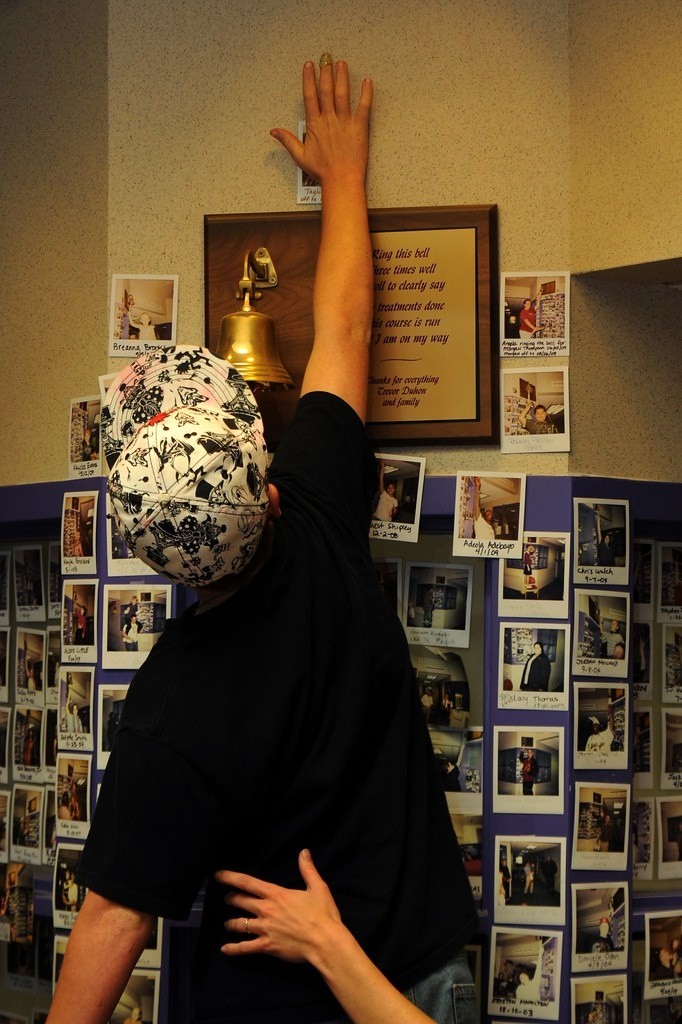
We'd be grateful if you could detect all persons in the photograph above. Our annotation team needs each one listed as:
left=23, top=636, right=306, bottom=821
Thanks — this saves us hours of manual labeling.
left=0, top=293, right=682, bottom=1024
left=41, top=52, right=480, bottom=1024
left=215, top=848, right=439, bottom=1024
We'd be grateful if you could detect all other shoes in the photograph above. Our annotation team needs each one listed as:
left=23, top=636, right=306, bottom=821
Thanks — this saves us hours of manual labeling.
left=424, top=620, right=431, bottom=625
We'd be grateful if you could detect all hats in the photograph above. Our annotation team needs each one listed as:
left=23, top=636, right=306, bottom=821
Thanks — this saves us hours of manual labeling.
left=100, top=345, right=269, bottom=588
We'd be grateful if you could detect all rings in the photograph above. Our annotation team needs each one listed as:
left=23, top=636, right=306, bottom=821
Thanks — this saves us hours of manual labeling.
left=245, top=918, right=251, bottom=933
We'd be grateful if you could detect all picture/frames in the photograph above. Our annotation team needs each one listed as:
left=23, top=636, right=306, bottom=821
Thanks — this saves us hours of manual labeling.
left=204, top=204, right=501, bottom=450
